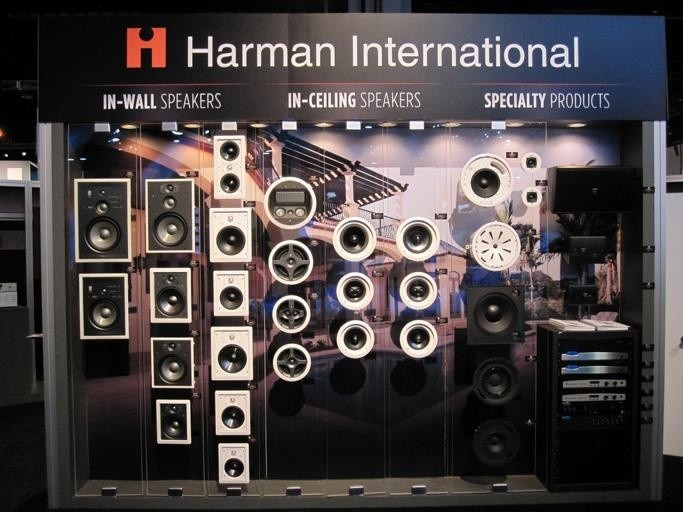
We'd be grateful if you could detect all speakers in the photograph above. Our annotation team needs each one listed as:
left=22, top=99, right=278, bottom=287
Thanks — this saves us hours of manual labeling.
left=453, top=154, right=537, bottom=478
left=74, top=178, right=132, bottom=262
left=78, top=272, right=130, bottom=341
left=265, top=176, right=448, bottom=477
left=144, top=178, right=195, bottom=446
left=535, top=166, right=644, bottom=493
left=208, top=135, right=254, bottom=488
